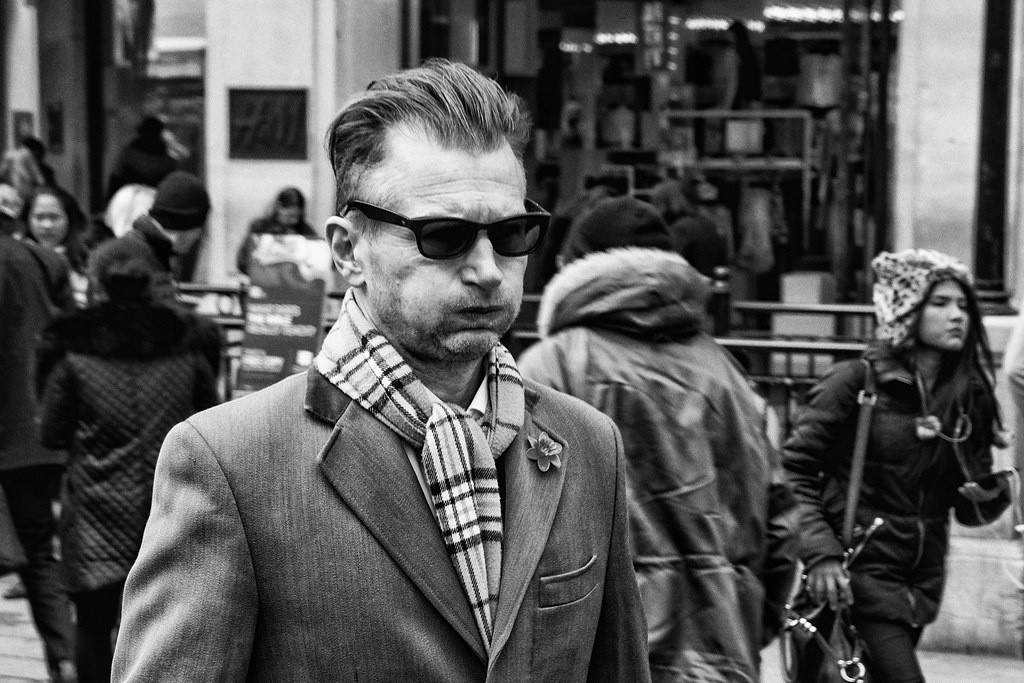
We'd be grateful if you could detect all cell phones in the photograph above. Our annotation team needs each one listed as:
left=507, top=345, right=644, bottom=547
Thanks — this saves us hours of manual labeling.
left=974, top=468, right=1021, bottom=490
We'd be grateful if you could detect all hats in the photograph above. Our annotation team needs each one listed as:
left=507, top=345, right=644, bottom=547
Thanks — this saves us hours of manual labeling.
left=563, top=196, right=672, bottom=263
left=136, top=115, right=163, bottom=139
left=872, top=249, right=1015, bottom=448
left=149, top=171, right=208, bottom=228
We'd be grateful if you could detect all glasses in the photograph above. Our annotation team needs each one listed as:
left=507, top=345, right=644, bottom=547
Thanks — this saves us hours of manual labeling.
left=336, top=198, right=552, bottom=260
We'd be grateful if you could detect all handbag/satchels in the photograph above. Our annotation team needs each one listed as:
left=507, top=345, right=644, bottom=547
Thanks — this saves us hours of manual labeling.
left=779, top=576, right=876, bottom=683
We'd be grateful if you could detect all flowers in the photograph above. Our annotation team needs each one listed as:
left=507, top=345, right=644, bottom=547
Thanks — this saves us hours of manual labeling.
left=523, top=431, right=563, bottom=472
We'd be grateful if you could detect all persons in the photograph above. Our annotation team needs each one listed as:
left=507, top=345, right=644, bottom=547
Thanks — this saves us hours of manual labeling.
left=236, top=189, right=314, bottom=276
left=652, top=183, right=728, bottom=335
left=109, top=62, right=652, bottom=683
left=513, top=195, right=802, bottom=683
left=0, top=116, right=221, bottom=683
left=782, top=249, right=1015, bottom=682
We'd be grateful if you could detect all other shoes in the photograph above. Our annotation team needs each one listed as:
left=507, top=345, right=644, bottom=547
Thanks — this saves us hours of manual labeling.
left=53, top=658, right=78, bottom=683
